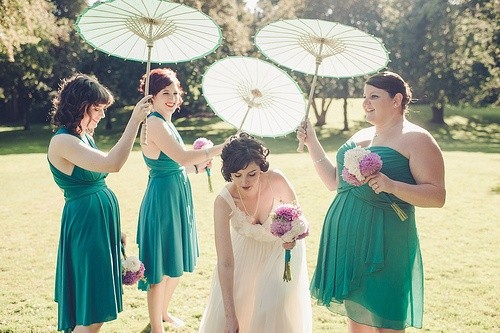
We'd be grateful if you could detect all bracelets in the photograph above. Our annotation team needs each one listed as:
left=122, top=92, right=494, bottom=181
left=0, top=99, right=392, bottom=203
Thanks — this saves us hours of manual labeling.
left=314, top=155, right=326, bottom=164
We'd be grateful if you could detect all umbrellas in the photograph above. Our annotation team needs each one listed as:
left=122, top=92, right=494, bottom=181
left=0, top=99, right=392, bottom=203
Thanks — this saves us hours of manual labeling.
left=200, top=55, right=307, bottom=139
left=73, top=0, right=225, bottom=145
left=251, top=18, right=391, bottom=153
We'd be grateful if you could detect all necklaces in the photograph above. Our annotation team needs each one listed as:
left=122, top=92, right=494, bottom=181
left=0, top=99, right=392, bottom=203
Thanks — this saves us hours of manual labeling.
left=374, top=134, right=379, bottom=137
left=237, top=181, right=261, bottom=217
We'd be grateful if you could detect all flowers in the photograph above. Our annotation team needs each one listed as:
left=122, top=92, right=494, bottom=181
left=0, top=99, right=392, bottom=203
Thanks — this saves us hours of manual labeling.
left=121, top=241, right=146, bottom=286
left=269, top=199, right=310, bottom=283
left=340, top=143, right=409, bottom=220
left=193, top=138, right=216, bottom=195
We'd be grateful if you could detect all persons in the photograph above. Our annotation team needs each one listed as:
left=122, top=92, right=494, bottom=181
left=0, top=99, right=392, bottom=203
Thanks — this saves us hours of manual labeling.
left=198, top=131, right=313, bottom=333
left=297, top=71, right=446, bottom=333
left=47, top=75, right=153, bottom=333
left=136, top=68, right=232, bottom=333
